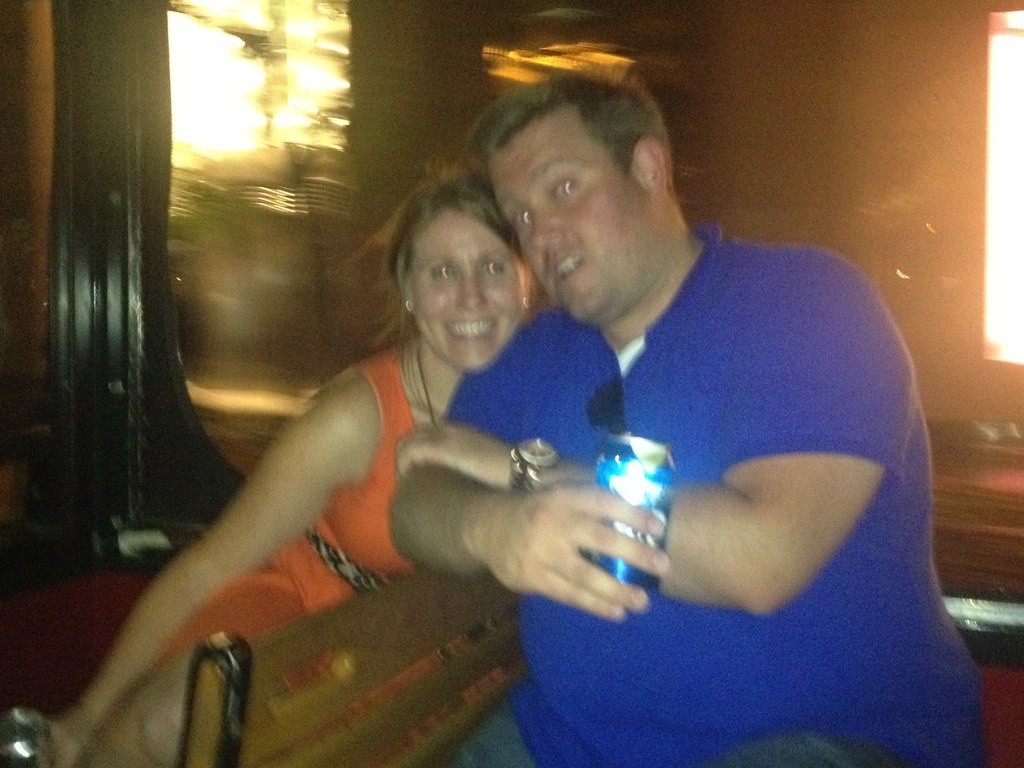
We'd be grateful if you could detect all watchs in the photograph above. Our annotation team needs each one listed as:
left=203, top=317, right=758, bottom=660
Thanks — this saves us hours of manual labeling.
left=509, top=438, right=559, bottom=492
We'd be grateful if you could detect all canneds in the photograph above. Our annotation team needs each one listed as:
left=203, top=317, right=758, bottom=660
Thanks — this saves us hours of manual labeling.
left=0, top=706, right=56, bottom=768
left=586, top=432, right=678, bottom=597
left=508, top=438, right=559, bottom=491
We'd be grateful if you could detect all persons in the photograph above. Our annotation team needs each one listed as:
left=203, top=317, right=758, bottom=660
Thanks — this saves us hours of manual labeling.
left=11, top=160, right=536, bottom=768
left=388, top=69, right=995, bottom=767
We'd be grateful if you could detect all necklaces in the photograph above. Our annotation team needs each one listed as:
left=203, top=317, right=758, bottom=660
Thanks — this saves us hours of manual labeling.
left=417, top=342, right=447, bottom=433
